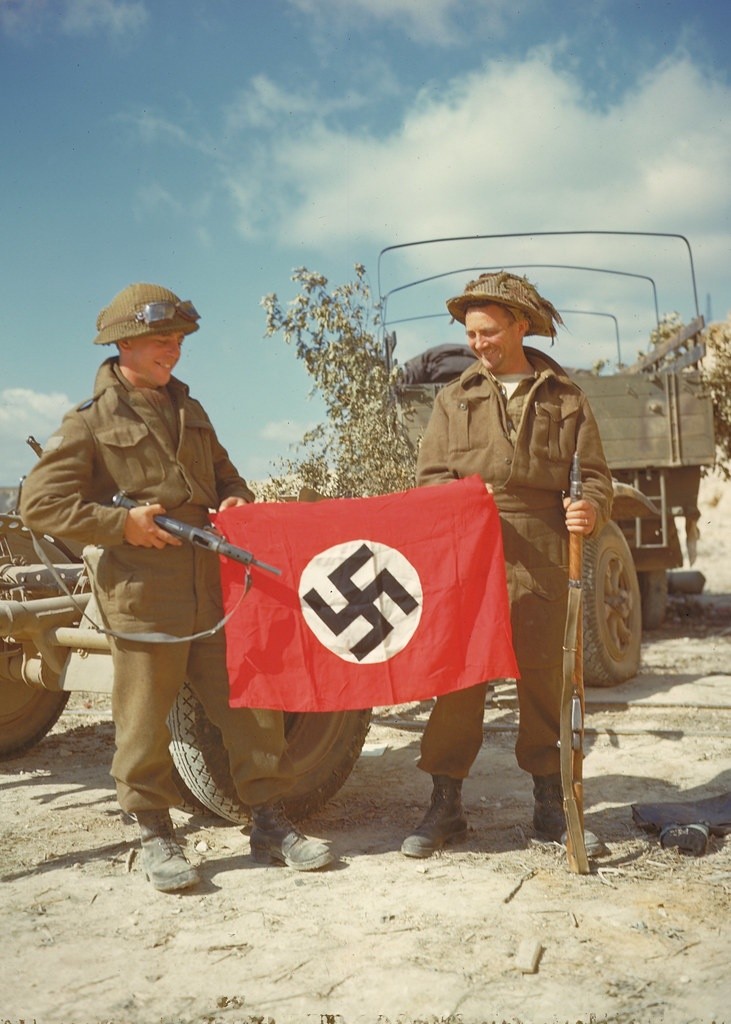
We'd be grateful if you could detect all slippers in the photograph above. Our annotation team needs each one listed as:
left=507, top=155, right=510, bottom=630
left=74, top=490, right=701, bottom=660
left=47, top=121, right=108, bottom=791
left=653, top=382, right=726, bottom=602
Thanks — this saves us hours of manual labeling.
left=532, top=775, right=602, bottom=856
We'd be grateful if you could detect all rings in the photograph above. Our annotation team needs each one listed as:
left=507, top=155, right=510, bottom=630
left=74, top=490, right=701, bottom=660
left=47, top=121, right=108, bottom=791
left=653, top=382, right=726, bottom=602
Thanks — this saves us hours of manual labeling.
left=585, top=518, right=588, bottom=525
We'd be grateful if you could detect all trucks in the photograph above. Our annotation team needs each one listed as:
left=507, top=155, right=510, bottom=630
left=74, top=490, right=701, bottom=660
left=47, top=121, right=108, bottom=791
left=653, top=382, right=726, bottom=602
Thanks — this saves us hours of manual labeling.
left=378, top=230, right=716, bottom=630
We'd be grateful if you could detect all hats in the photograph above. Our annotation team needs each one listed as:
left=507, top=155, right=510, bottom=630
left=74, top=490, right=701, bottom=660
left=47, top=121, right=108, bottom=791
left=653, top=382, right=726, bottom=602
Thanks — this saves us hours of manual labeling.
left=93, top=284, right=200, bottom=344
left=446, top=271, right=556, bottom=337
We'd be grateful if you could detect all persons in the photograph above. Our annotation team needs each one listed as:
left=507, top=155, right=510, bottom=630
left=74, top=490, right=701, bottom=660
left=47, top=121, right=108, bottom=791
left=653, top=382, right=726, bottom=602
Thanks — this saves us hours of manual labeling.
left=401, top=270, right=614, bottom=858
left=21, top=284, right=334, bottom=890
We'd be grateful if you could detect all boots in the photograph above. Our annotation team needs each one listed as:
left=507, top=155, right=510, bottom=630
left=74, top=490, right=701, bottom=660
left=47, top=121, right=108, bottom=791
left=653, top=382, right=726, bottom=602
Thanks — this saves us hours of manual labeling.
left=134, top=809, right=200, bottom=890
left=401, top=774, right=467, bottom=858
left=249, top=797, right=334, bottom=871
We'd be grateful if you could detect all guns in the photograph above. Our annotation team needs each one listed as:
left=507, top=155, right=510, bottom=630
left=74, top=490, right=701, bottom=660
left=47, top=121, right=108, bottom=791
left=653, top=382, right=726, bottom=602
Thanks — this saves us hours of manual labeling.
left=556, top=450, right=591, bottom=876
left=15, top=435, right=284, bottom=578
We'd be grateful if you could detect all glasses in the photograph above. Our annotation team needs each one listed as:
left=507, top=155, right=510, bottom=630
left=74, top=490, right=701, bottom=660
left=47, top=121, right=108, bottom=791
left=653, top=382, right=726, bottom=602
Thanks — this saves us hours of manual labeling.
left=101, top=300, right=201, bottom=331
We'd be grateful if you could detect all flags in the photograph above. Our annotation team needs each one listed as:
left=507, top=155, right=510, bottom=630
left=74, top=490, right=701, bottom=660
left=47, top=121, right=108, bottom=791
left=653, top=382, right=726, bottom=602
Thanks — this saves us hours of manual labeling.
left=207, top=472, right=522, bottom=713
